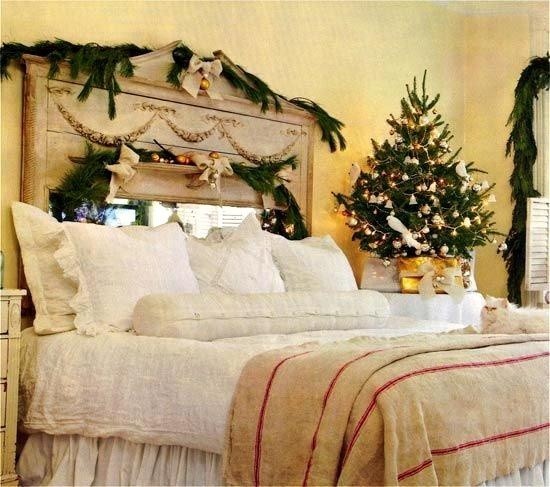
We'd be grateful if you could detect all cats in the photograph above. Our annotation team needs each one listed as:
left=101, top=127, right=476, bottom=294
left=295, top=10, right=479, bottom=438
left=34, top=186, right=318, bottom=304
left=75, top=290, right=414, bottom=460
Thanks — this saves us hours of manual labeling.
left=481, top=294, right=550, bottom=334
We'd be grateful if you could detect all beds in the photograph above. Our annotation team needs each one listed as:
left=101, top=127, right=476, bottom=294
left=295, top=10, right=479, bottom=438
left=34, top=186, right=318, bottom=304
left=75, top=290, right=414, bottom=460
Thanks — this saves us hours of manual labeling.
left=13, top=300, right=550, bottom=485
